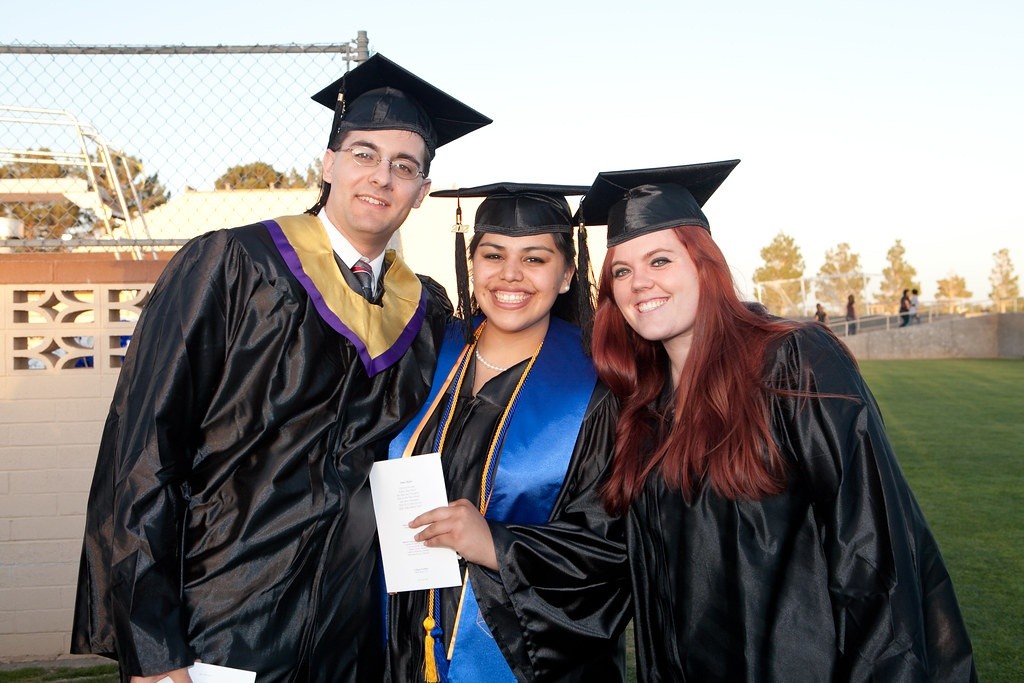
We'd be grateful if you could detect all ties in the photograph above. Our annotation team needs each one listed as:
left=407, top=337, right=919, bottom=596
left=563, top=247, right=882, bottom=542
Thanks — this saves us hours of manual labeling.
left=353, top=260, right=373, bottom=302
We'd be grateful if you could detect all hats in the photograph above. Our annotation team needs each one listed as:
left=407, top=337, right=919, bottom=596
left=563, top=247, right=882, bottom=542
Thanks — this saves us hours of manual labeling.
left=572, top=158, right=741, bottom=358
left=429, top=182, right=591, bottom=346
left=305, top=51, right=493, bottom=217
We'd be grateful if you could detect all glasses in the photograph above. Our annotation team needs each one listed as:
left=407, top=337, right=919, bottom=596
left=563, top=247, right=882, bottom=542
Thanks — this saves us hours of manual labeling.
left=330, top=146, right=426, bottom=179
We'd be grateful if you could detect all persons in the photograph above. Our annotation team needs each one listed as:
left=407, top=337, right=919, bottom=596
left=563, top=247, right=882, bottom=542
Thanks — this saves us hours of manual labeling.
left=71, top=52, right=454, bottom=683
left=815, top=288, right=921, bottom=336
left=570, top=159, right=979, bottom=683
left=388, top=182, right=637, bottom=683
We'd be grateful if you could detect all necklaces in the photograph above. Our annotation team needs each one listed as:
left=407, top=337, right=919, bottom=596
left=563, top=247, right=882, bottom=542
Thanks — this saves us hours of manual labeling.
left=473, top=348, right=509, bottom=375
